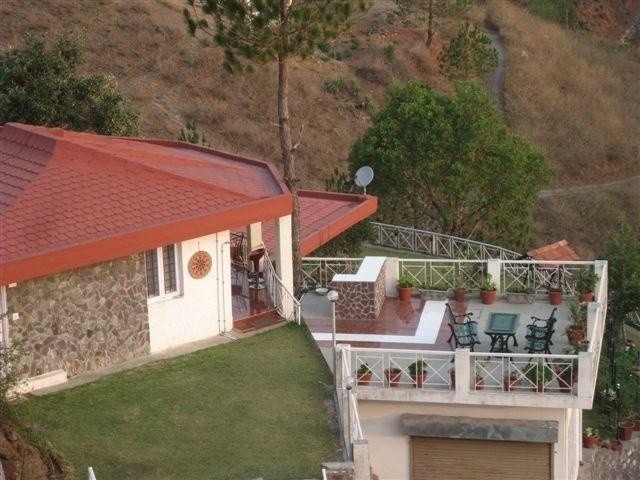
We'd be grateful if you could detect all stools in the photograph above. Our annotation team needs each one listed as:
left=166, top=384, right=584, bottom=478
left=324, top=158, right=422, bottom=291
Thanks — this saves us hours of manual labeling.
left=247, top=248, right=265, bottom=290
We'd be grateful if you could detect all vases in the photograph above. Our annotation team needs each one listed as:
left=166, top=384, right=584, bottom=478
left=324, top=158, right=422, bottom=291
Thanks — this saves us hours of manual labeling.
left=354, top=359, right=430, bottom=389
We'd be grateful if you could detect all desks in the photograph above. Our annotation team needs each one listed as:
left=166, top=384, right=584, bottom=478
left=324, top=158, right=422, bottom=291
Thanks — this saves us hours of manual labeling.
left=483, top=309, right=521, bottom=360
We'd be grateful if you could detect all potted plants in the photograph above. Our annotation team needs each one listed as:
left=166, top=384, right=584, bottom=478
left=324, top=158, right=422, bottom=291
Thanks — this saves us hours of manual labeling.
left=548, top=268, right=600, bottom=394
left=448, top=365, right=484, bottom=391
left=452, top=272, right=498, bottom=305
left=395, top=269, right=416, bottom=302
left=506, top=285, right=537, bottom=305
left=583, top=411, right=640, bottom=456
left=501, top=359, right=552, bottom=393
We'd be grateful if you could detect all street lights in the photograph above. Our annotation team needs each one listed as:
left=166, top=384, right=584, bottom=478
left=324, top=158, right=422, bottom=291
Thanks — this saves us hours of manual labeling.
left=341, top=373, right=356, bottom=462
left=325, top=288, right=339, bottom=397
left=607, top=315, right=622, bottom=440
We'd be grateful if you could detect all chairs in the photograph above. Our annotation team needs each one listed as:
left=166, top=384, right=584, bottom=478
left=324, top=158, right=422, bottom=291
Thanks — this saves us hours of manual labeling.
left=441, top=301, right=482, bottom=364
left=523, top=308, right=559, bottom=368
